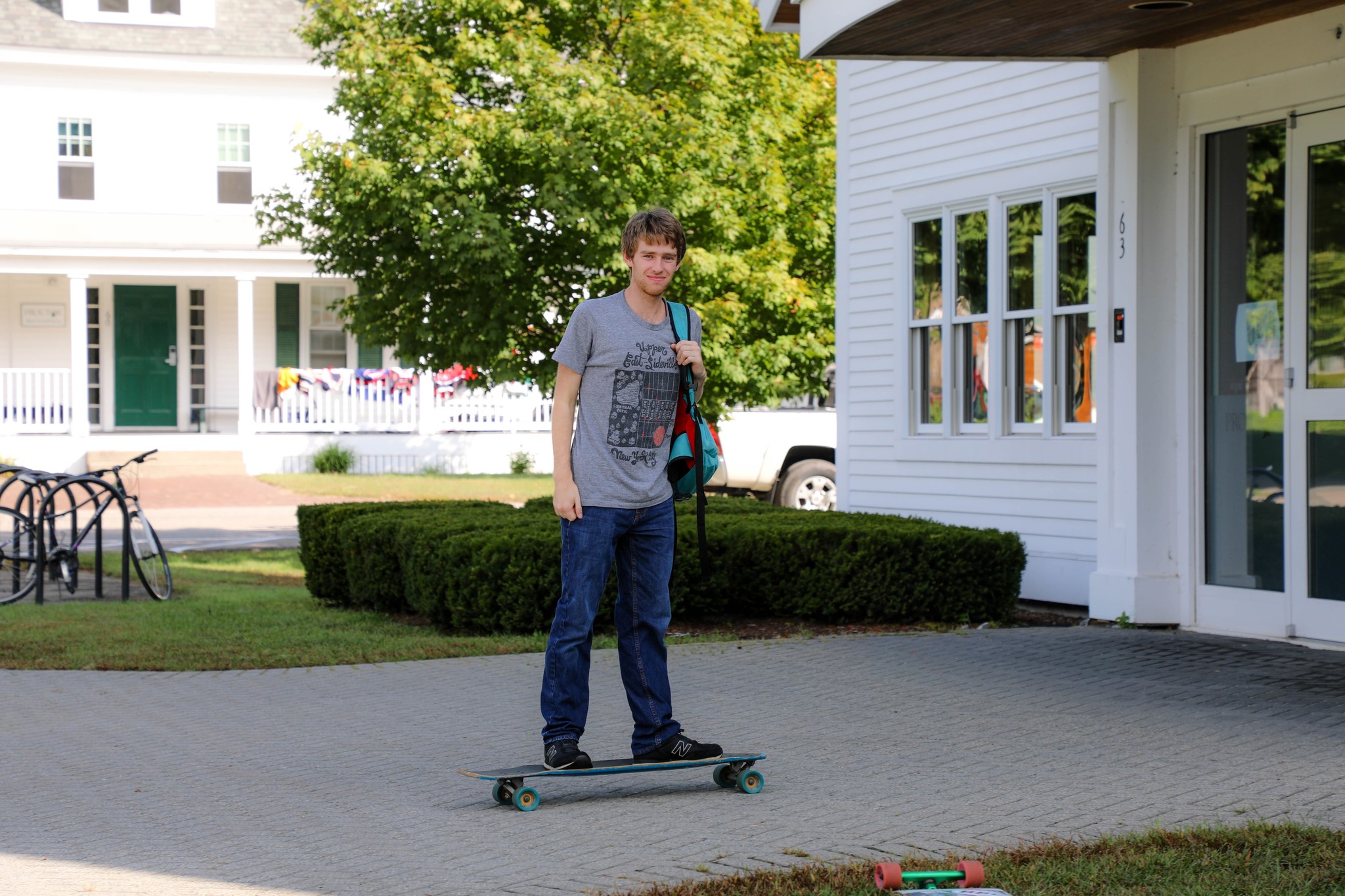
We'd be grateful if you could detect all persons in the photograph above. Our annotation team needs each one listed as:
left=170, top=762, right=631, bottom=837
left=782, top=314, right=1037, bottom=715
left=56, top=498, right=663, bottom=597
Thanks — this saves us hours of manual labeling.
left=537, top=211, right=723, bottom=766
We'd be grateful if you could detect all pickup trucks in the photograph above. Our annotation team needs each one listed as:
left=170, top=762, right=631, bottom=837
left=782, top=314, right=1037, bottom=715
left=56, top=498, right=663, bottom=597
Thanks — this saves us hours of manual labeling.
left=706, top=363, right=838, bottom=514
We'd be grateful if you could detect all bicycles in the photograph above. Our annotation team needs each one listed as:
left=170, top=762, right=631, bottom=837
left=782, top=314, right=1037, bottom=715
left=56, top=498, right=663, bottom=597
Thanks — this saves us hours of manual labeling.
left=0, top=449, right=172, bottom=608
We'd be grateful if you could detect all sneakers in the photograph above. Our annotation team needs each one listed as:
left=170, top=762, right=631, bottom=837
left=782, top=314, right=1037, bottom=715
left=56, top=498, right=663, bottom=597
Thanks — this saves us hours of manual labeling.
left=633, top=729, right=723, bottom=765
left=544, top=739, right=594, bottom=770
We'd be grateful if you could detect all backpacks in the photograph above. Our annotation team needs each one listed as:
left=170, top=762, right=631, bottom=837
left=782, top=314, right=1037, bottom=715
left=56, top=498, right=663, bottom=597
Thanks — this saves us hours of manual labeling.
left=664, top=300, right=719, bottom=501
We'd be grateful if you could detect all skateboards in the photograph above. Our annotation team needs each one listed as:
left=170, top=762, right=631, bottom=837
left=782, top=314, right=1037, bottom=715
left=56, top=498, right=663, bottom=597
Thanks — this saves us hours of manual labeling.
left=457, top=751, right=766, bottom=811
left=873, top=858, right=1010, bottom=896
